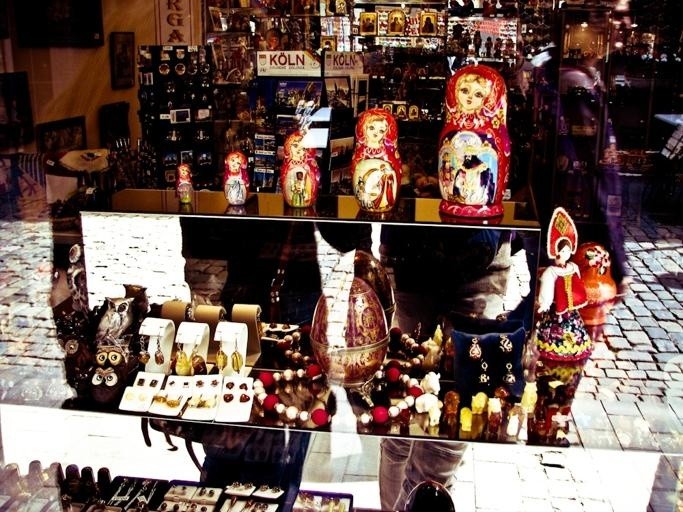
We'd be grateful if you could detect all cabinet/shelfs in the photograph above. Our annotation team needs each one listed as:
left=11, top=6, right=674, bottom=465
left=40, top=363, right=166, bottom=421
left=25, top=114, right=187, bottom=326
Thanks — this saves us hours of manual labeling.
left=548, top=6, right=663, bottom=226
left=321, top=74, right=370, bottom=208
left=271, top=77, right=330, bottom=210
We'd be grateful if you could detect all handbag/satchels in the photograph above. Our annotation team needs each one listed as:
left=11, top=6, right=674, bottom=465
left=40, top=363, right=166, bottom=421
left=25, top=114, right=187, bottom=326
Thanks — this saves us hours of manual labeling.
left=141, top=415, right=253, bottom=455
left=549, top=172, right=603, bottom=226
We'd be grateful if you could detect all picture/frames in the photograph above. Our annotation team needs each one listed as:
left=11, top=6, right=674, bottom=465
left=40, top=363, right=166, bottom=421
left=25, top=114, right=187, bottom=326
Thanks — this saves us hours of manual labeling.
left=36, top=116, right=87, bottom=153
left=383, top=104, right=393, bottom=115
left=419, top=12, right=438, bottom=36
left=0, top=71, right=34, bottom=148
left=109, top=32, right=135, bottom=90
left=409, top=105, right=419, bottom=120
left=14, top=0, right=104, bottom=49
left=397, top=105, right=406, bottom=119
left=208, top=6, right=223, bottom=31
left=320, top=36, right=337, bottom=52
left=388, top=9, right=406, bottom=37
left=360, top=12, right=378, bottom=36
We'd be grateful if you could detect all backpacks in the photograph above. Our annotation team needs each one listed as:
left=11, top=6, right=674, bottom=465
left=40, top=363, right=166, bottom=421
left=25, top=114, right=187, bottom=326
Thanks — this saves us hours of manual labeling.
left=380, top=223, right=500, bottom=296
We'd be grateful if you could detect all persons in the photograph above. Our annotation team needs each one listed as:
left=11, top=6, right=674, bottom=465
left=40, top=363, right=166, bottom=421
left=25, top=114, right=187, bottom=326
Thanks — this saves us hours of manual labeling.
left=371, top=224, right=516, bottom=506
left=180, top=214, right=341, bottom=489
left=524, top=0, right=637, bottom=350
left=539, top=204, right=589, bottom=326
